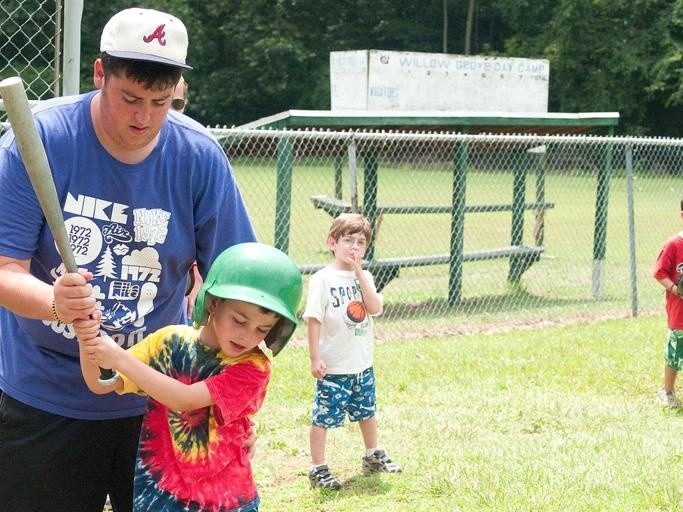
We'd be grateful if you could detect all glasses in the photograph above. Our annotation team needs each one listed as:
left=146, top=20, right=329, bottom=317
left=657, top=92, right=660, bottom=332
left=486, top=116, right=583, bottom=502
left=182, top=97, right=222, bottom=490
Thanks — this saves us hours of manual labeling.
left=170, top=98, right=186, bottom=111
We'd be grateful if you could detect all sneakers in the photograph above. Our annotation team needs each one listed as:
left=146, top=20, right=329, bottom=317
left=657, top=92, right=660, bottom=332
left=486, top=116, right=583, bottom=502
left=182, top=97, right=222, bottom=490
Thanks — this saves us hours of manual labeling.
left=359, top=447, right=402, bottom=474
left=307, top=465, right=342, bottom=490
left=657, top=385, right=681, bottom=408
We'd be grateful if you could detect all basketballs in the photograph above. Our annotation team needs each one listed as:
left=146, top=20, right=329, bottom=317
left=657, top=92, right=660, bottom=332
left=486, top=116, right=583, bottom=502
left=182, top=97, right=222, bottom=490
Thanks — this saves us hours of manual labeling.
left=347, top=300, right=366, bottom=324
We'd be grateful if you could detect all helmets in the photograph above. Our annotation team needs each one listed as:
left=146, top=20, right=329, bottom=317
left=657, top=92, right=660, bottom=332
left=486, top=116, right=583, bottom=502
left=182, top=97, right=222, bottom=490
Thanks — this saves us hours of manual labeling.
left=191, top=240, right=304, bottom=358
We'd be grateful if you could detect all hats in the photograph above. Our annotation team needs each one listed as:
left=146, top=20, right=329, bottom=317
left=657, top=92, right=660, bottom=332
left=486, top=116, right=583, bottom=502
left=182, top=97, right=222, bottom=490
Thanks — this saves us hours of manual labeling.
left=99, top=9, right=194, bottom=71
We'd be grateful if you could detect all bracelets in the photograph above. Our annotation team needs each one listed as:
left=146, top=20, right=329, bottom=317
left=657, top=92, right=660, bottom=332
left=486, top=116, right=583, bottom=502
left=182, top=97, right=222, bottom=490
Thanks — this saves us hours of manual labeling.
left=668, top=282, right=675, bottom=293
left=49, top=295, right=67, bottom=327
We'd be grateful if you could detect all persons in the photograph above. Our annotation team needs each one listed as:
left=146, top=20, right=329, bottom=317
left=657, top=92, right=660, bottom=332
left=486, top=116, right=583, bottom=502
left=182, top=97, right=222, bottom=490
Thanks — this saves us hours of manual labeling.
left=651, top=195, right=683, bottom=410
left=0, top=6, right=262, bottom=512
left=70, top=239, right=306, bottom=512
left=168, top=71, right=206, bottom=324
left=298, top=211, right=403, bottom=491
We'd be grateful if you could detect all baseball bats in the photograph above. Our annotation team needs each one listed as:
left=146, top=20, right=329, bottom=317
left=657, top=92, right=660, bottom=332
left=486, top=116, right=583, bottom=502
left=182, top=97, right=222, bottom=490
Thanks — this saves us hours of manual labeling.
left=0, top=76, right=118, bottom=385
